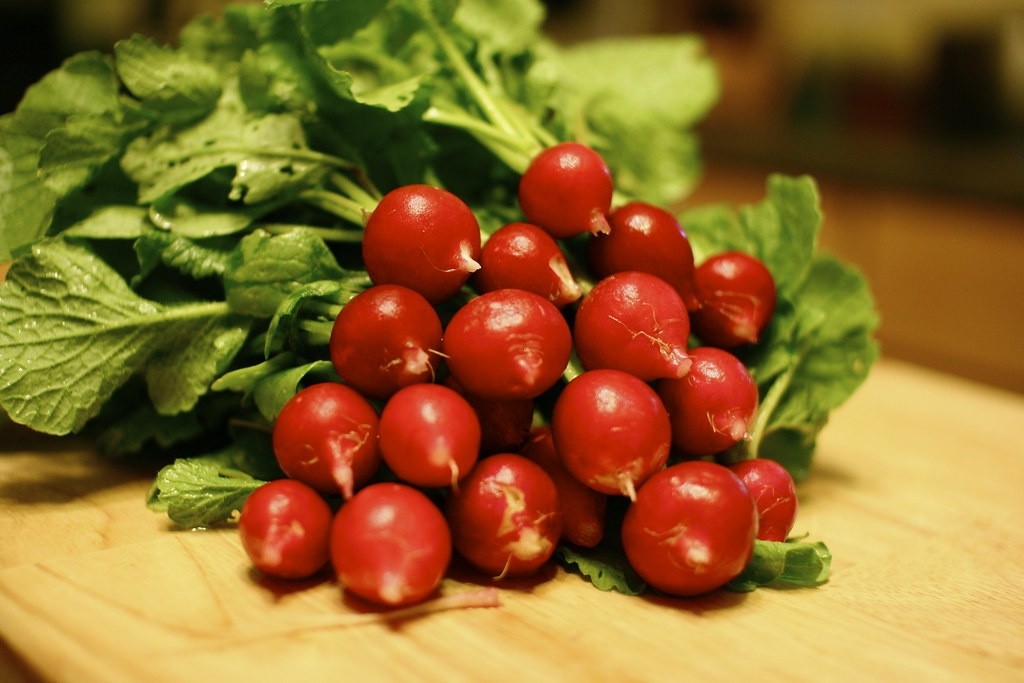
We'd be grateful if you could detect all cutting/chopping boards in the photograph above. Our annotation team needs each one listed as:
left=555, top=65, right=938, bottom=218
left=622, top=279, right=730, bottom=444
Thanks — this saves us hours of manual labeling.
left=0, top=349, right=1024, bottom=683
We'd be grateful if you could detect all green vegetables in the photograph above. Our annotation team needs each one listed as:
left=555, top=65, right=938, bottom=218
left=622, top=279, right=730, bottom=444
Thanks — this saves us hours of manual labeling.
left=0, top=0, right=879, bottom=595
